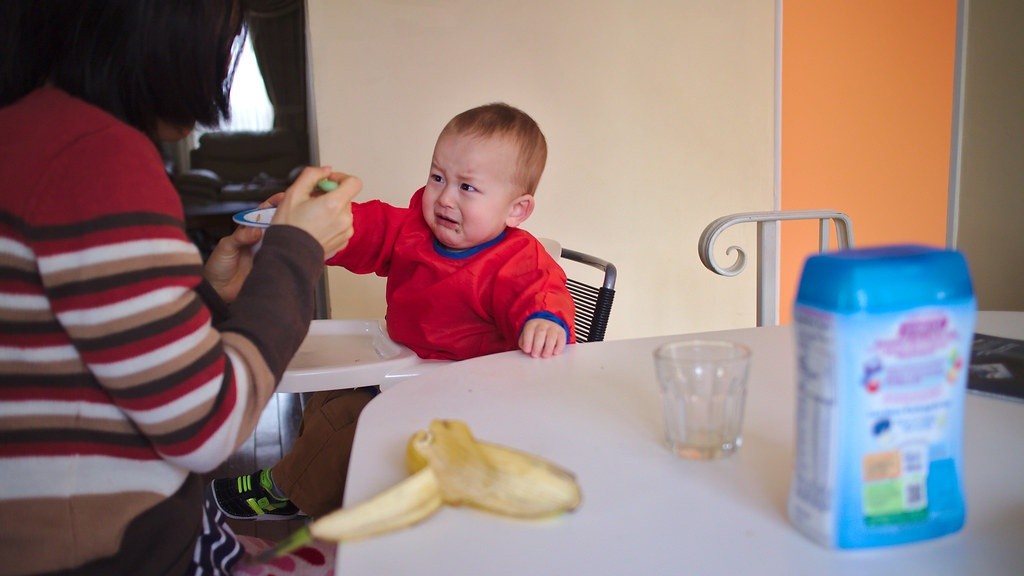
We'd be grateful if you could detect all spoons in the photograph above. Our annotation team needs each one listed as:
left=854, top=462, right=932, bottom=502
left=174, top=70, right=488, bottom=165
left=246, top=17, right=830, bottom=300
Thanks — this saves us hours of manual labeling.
left=289, top=166, right=338, bottom=193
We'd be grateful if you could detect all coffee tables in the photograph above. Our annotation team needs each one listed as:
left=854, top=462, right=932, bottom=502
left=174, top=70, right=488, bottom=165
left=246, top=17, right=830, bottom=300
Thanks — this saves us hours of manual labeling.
left=182, top=201, right=261, bottom=263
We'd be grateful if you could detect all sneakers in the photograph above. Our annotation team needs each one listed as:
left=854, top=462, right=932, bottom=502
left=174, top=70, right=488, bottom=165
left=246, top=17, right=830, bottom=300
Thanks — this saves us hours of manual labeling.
left=211, top=466, right=302, bottom=521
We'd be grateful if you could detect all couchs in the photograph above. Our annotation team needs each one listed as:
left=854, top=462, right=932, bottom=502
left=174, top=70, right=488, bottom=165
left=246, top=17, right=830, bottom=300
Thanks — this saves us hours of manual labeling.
left=173, top=131, right=274, bottom=238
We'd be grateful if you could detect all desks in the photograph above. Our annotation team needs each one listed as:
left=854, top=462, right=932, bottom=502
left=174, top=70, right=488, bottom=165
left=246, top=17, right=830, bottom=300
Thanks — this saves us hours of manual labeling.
left=333, top=312, right=1023, bottom=576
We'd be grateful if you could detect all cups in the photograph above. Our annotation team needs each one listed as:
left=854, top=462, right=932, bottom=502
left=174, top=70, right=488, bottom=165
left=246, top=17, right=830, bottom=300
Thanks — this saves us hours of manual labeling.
left=653, top=338, right=753, bottom=461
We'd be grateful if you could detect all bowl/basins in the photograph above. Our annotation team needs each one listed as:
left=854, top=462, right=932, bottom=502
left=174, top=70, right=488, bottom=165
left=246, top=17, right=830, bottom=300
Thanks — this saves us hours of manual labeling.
left=232, top=206, right=278, bottom=260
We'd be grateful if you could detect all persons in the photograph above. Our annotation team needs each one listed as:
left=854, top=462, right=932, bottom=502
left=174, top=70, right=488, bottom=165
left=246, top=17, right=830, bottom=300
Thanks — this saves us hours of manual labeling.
left=211, top=103, right=575, bottom=522
left=0, top=0, right=362, bottom=576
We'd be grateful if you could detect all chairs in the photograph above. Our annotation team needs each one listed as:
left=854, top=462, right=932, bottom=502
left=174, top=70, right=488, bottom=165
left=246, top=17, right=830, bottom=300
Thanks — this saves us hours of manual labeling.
left=278, top=233, right=619, bottom=392
